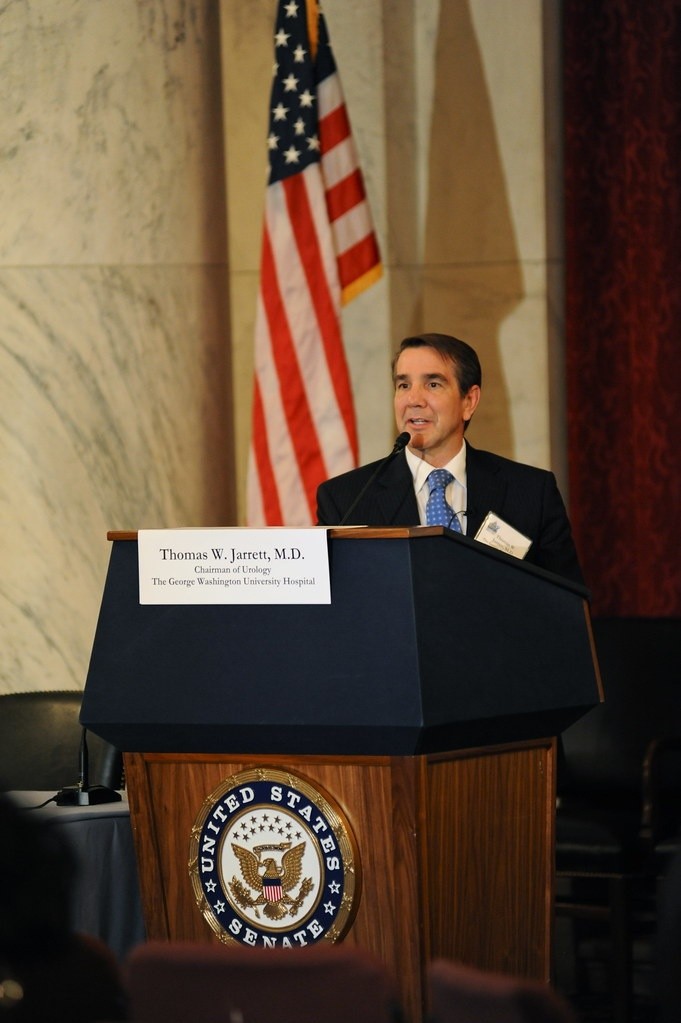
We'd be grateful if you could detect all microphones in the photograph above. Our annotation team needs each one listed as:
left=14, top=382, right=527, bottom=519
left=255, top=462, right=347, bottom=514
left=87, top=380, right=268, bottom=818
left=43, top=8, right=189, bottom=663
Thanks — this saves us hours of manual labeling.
left=337, top=431, right=411, bottom=525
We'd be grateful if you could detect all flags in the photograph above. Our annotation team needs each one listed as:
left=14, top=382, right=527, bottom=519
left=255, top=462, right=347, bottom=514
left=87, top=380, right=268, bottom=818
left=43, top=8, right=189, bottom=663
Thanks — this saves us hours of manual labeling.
left=239, top=0, right=385, bottom=528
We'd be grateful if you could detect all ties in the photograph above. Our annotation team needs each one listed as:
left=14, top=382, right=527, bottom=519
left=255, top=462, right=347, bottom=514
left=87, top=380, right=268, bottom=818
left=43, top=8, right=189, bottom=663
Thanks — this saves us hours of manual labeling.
left=426, top=469, right=464, bottom=536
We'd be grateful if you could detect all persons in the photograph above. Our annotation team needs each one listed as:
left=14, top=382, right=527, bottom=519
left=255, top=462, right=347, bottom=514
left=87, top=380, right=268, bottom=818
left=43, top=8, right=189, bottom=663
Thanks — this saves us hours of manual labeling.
left=0, top=790, right=126, bottom=1023
left=315, top=333, right=582, bottom=576
